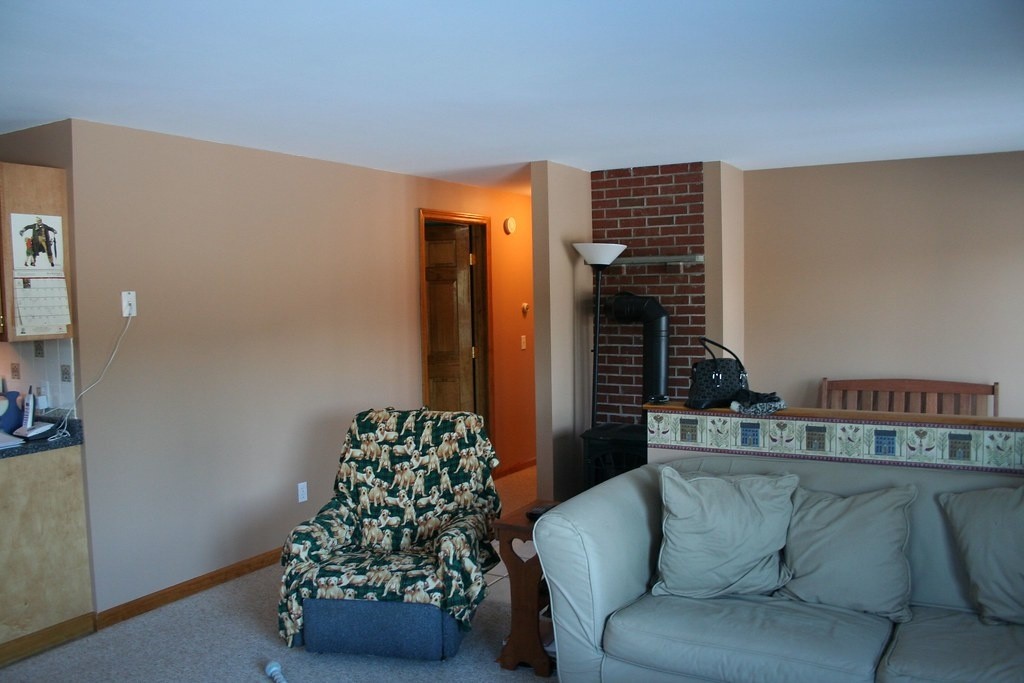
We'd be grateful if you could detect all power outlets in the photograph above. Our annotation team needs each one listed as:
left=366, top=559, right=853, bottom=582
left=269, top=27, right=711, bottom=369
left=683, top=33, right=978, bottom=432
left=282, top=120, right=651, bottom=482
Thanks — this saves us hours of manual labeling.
left=40, top=381, right=51, bottom=408
left=298, top=482, right=308, bottom=503
left=121, top=291, right=138, bottom=317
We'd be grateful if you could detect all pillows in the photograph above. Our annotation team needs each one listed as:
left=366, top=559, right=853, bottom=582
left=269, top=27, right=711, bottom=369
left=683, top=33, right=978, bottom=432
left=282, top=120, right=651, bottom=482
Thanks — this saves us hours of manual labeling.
left=938, top=484, right=1024, bottom=625
left=651, top=463, right=801, bottom=599
left=771, top=484, right=918, bottom=624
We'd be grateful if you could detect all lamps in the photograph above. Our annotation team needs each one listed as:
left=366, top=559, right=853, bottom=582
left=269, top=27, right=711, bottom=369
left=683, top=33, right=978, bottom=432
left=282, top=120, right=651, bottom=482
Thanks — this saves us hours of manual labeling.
left=572, top=242, right=628, bottom=430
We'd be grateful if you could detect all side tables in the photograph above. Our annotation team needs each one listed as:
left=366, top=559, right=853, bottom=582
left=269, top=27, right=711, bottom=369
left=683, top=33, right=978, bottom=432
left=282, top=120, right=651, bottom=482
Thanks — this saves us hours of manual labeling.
left=494, top=500, right=565, bottom=677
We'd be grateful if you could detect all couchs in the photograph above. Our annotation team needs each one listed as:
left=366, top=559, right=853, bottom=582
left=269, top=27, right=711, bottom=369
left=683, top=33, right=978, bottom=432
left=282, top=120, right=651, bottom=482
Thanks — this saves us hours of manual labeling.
left=533, top=456, right=1024, bottom=683
left=278, top=406, right=502, bottom=663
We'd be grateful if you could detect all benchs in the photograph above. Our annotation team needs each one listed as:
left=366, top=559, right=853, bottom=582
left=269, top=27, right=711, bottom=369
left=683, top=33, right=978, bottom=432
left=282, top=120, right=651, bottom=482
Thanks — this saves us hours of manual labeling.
left=820, top=378, right=1000, bottom=417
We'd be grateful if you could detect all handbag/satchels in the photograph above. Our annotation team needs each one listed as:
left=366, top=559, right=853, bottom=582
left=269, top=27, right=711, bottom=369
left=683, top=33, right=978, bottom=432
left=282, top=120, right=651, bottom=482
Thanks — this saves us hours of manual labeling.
left=684, top=337, right=749, bottom=410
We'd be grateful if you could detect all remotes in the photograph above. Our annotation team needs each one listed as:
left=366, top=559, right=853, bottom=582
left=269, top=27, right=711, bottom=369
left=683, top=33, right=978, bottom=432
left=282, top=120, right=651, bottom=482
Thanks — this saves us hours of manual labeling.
left=526, top=504, right=556, bottom=523
